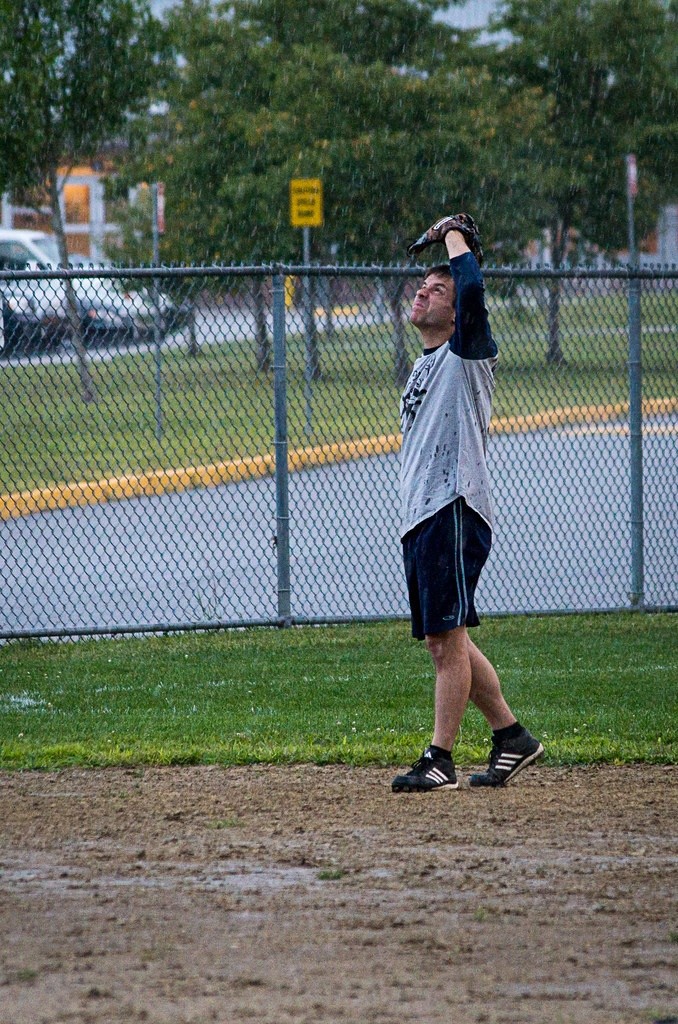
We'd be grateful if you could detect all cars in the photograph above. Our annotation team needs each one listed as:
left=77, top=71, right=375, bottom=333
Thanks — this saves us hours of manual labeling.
left=0, top=226, right=195, bottom=355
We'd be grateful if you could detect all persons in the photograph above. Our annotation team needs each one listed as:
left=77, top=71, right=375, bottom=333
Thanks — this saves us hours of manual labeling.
left=390, top=213, right=544, bottom=793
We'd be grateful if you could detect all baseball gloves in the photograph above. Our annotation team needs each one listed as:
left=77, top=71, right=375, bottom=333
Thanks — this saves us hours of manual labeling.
left=405, top=212, right=483, bottom=267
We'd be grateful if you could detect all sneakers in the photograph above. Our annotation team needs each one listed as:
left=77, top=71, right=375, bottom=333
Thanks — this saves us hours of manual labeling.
left=391, top=748, right=458, bottom=793
left=469, top=727, right=545, bottom=787
